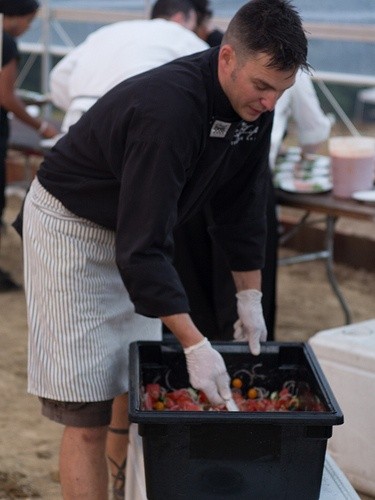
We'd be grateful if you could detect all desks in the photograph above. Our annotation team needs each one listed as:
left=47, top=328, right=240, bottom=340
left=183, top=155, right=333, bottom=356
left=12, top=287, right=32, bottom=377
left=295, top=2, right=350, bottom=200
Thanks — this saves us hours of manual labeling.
left=8, top=115, right=375, bottom=325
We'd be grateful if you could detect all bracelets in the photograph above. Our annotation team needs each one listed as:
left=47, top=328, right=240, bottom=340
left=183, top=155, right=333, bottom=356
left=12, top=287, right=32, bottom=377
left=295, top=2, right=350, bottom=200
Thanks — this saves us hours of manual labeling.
left=38, top=122, right=48, bottom=135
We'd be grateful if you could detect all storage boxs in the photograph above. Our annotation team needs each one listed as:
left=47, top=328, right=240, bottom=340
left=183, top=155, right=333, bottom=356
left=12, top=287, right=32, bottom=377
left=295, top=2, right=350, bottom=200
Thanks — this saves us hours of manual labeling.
left=125, top=319, right=375, bottom=500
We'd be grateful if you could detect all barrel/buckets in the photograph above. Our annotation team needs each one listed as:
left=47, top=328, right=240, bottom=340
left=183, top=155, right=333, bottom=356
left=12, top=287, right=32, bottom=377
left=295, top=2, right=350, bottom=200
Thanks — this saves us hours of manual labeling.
left=329, top=136, right=375, bottom=199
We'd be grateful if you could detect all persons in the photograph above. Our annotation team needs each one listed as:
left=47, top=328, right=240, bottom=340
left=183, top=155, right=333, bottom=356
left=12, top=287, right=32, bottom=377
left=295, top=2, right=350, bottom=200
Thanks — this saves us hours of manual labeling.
left=40, top=0, right=211, bottom=148
left=192, top=0, right=224, bottom=48
left=23, top=0, right=313, bottom=500
left=0, top=0, right=59, bottom=292
left=162, top=67, right=330, bottom=342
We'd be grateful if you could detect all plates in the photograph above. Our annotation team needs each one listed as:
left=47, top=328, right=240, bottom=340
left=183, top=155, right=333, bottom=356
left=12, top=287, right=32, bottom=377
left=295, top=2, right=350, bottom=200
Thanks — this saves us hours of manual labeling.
left=352, top=189, right=374, bottom=201
left=272, top=149, right=334, bottom=195
